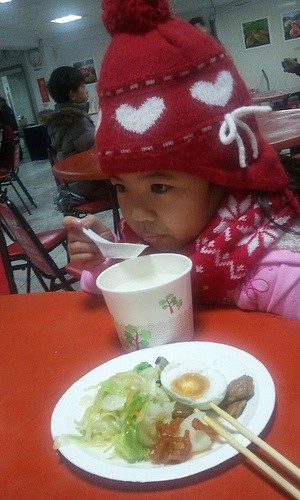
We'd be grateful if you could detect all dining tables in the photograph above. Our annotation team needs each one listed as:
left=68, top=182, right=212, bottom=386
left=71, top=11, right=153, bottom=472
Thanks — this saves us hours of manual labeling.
left=0, top=292, right=300, bottom=500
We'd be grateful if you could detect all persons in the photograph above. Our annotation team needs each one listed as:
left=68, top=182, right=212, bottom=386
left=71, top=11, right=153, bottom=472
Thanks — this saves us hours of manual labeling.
left=41, top=66, right=117, bottom=204
left=0, top=96, right=18, bottom=176
left=281, top=58, right=300, bottom=77
left=188, top=16, right=208, bottom=35
left=61, top=0, right=300, bottom=321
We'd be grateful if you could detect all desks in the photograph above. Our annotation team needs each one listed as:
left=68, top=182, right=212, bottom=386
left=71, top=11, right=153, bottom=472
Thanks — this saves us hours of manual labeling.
left=53, top=106, right=300, bottom=180
left=247, top=86, right=300, bottom=106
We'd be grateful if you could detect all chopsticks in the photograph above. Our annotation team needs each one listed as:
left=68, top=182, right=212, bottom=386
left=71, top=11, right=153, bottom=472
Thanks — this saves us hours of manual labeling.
left=204, top=402, right=300, bottom=500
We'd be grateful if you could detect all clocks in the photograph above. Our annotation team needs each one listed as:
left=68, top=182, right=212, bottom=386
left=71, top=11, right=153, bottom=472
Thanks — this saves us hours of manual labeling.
left=28, top=50, right=43, bottom=67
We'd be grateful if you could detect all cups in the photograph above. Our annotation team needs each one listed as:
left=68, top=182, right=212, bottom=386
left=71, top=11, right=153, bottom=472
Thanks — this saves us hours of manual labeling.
left=95, top=252, right=193, bottom=353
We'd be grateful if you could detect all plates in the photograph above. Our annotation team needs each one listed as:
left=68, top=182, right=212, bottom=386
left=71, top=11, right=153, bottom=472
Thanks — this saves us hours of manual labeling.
left=50, top=341, right=276, bottom=484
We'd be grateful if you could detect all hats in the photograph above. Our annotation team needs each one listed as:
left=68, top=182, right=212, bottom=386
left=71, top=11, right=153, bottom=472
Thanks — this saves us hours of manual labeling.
left=95, top=0, right=299, bottom=214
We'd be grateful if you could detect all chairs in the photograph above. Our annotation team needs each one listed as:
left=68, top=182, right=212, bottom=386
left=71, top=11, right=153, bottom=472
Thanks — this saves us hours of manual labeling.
left=49, top=146, right=124, bottom=237
left=0, top=198, right=70, bottom=293
left=0, top=141, right=37, bottom=215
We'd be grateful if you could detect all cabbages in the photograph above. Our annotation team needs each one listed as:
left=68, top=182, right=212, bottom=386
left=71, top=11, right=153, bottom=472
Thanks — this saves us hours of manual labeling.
left=53, top=360, right=216, bottom=463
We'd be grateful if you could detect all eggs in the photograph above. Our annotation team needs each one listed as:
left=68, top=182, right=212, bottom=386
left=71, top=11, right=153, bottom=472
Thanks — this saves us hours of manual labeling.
left=160, top=357, right=226, bottom=412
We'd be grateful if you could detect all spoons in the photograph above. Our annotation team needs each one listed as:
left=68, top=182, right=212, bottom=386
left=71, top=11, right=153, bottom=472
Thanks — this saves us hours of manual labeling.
left=77, top=226, right=149, bottom=260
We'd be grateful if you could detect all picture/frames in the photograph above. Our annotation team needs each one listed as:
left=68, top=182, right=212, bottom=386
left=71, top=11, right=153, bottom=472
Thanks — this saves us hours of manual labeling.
left=241, top=16, right=272, bottom=51
left=72, top=57, right=98, bottom=86
left=280, top=8, right=300, bottom=42
